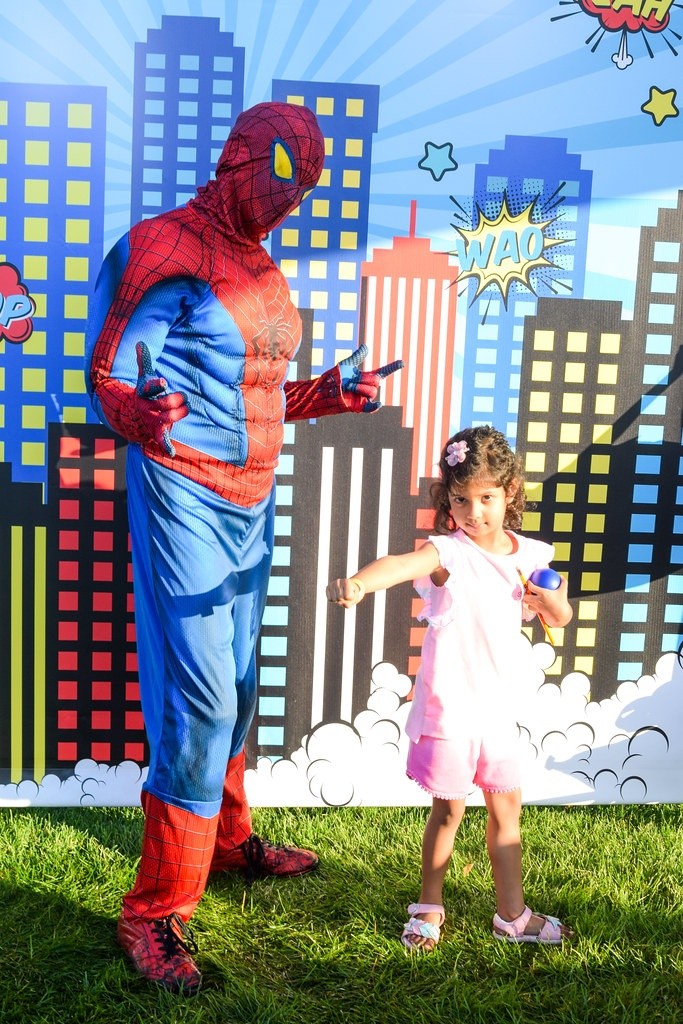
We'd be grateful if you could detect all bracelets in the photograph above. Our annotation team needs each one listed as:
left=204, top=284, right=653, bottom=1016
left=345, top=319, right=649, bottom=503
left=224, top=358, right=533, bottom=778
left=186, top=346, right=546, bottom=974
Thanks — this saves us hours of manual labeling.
left=350, top=575, right=366, bottom=604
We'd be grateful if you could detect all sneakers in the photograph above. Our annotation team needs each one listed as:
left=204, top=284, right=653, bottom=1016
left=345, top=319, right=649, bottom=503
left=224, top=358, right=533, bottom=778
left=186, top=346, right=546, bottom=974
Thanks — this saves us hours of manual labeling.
left=211, top=834, right=318, bottom=886
left=117, top=907, right=202, bottom=994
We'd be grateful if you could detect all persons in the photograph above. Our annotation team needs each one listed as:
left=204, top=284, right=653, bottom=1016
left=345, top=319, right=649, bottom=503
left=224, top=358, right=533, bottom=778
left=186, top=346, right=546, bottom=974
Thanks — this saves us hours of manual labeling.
left=326, top=425, right=576, bottom=954
left=89, top=101, right=402, bottom=989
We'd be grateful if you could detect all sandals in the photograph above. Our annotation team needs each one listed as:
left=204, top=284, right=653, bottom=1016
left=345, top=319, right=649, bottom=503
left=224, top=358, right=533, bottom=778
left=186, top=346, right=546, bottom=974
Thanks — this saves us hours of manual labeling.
left=492, top=906, right=577, bottom=945
left=401, top=902, right=445, bottom=954
